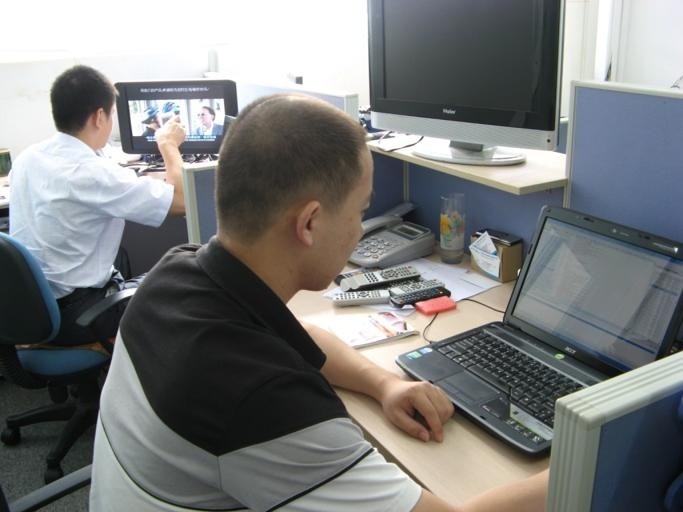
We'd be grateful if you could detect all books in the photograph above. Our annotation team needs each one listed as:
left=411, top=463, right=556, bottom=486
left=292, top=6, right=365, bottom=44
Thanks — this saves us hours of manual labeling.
left=302, top=309, right=420, bottom=349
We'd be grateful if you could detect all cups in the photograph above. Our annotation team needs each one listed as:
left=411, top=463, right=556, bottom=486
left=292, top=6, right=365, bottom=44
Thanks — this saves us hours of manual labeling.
left=439, top=194, right=467, bottom=265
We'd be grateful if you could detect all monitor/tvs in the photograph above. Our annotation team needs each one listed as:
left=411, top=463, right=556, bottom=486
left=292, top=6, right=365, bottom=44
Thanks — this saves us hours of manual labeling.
left=367, top=0, right=566, bottom=166
left=114, top=80, right=239, bottom=166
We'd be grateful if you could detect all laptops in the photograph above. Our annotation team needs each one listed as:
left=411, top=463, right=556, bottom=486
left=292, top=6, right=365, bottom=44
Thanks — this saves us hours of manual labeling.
left=394, top=204, right=683, bottom=457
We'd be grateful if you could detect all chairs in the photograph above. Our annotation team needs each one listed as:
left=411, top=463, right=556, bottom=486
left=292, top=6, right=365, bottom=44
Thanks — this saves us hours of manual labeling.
left=1, top=231, right=141, bottom=484
left=0, top=465, right=92, bottom=511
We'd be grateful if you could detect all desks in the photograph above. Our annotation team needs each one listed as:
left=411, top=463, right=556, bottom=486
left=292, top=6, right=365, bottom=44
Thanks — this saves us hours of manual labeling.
left=181, top=241, right=681, bottom=511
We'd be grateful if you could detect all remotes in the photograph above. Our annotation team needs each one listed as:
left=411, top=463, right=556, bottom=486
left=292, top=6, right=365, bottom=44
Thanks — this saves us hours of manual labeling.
left=390, top=286, right=451, bottom=309
left=389, top=278, right=445, bottom=296
left=340, top=265, right=421, bottom=291
left=333, top=266, right=378, bottom=283
left=332, top=289, right=390, bottom=306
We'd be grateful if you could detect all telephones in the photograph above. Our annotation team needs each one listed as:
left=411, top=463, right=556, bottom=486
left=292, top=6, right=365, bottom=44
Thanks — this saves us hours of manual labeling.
left=351, top=214, right=436, bottom=267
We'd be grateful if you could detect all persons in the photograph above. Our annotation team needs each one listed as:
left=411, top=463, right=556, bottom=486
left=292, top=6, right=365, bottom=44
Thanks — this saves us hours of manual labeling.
left=89, top=93, right=549, bottom=511
left=140, top=101, right=223, bottom=136
left=9, top=65, right=186, bottom=346
